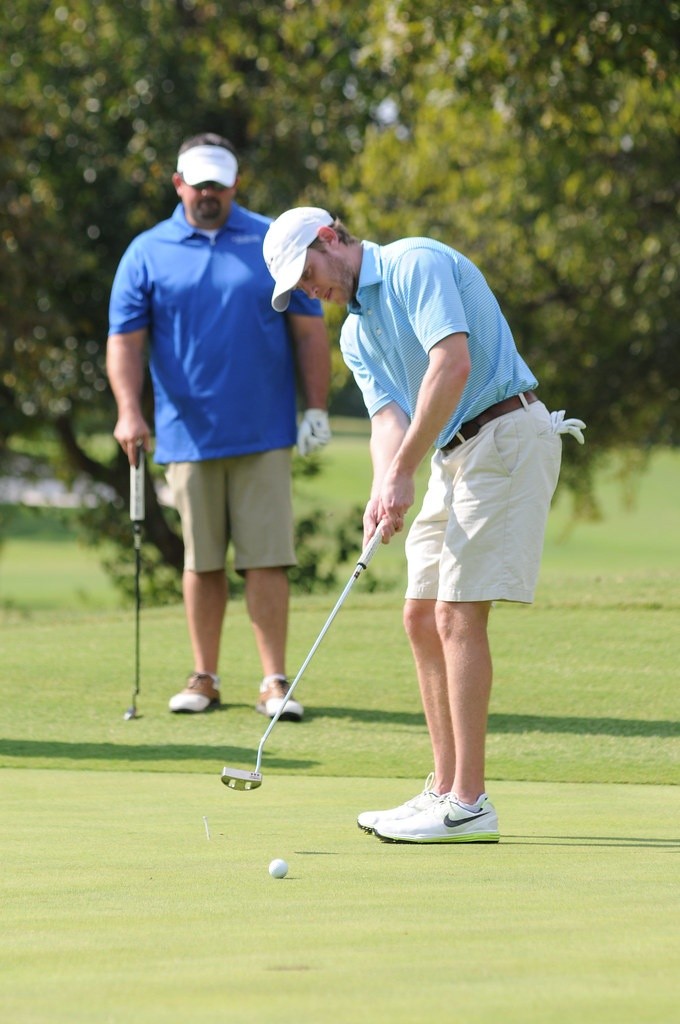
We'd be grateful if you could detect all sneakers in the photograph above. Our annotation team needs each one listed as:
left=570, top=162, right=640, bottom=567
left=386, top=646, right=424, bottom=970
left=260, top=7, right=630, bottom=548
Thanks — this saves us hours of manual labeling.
left=371, top=791, right=503, bottom=843
left=166, top=673, right=223, bottom=713
left=357, top=773, right=442, bottom=835
left=256, top=676, right=308, bottom=723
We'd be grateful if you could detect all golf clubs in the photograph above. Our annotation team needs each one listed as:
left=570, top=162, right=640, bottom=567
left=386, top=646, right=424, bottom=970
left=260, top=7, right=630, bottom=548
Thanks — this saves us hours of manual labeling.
left=221, top=519, right=382, bottom=791
left=122, top=437, right=145, bottom=720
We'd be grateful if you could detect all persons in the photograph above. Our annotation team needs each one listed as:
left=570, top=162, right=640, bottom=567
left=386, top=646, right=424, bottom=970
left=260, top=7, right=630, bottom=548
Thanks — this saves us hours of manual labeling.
left=264, top=206, right=561, bottom=844
left=103, top=133, right=332, bottom=718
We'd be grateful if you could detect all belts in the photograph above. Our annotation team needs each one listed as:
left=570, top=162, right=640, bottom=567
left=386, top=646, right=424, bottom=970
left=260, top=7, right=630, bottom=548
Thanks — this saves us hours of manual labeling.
left=438, top=391, right=538, bottom=454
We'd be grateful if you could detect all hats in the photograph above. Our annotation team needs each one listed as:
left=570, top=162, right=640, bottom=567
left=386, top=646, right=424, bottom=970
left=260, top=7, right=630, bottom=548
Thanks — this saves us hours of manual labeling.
left=173, top=144, right=240, bottom=187
left=260, top=206, right=333, bottom=312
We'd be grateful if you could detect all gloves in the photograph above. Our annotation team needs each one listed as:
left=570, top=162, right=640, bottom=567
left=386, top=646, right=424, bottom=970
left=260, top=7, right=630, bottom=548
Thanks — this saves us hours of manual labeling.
left=296, top=408, right=335, bottom=459
left=552, top=408, right=590, bottom=444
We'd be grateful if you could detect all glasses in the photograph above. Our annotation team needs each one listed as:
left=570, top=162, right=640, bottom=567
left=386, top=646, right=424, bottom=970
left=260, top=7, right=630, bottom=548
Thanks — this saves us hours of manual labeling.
left=193, top=180, right=227, bottom=192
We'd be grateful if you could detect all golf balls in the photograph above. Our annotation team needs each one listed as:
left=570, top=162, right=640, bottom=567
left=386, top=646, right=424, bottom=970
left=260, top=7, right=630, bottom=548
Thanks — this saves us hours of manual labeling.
left=269, top=858, right=288, bottom=879
